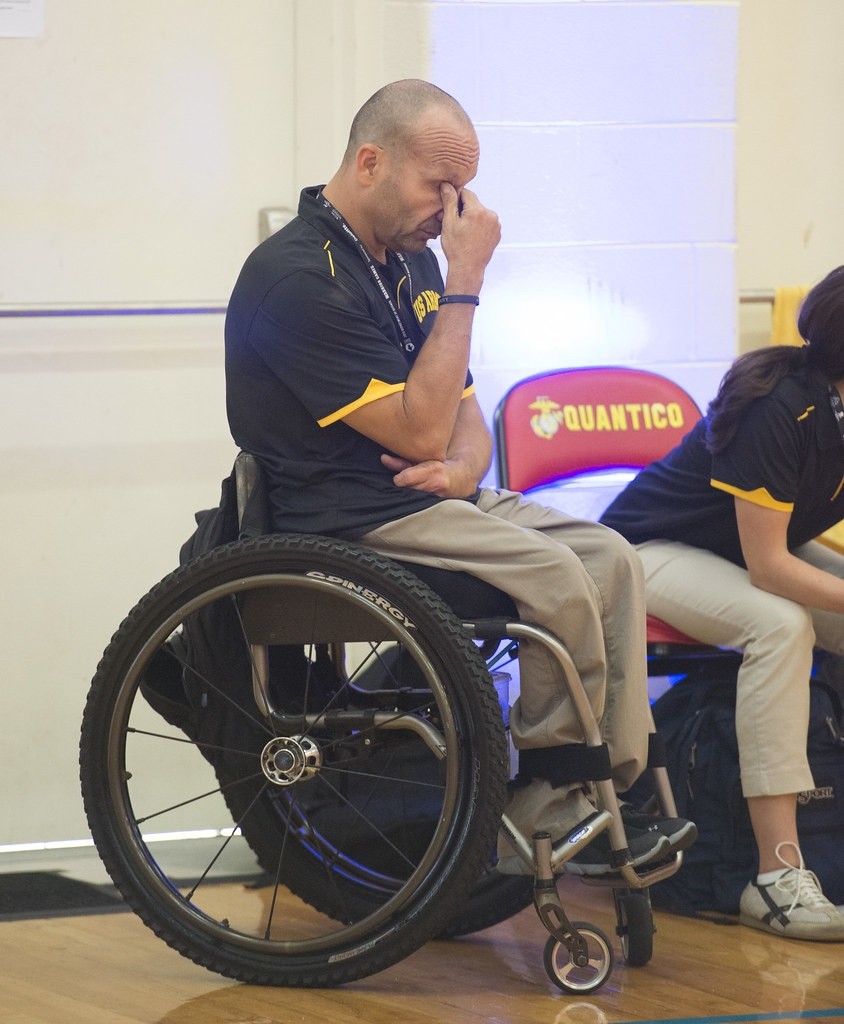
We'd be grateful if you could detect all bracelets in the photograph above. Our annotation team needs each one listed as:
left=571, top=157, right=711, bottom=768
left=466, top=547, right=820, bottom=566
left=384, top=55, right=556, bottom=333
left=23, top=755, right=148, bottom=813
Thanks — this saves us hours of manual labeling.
left=438, top=295, right=479, bottom=307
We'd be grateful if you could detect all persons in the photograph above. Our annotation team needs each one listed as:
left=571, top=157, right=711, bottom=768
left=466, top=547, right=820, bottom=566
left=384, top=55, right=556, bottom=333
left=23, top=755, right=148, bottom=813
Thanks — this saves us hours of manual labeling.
left=225, top=79, right=698, bottom=876
left=597, top=265, right=844, bottom=941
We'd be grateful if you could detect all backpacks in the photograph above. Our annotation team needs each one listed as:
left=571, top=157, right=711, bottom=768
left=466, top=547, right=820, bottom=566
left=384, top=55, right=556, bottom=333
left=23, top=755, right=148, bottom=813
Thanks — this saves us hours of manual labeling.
left=619, top=670, right=844, bottom=925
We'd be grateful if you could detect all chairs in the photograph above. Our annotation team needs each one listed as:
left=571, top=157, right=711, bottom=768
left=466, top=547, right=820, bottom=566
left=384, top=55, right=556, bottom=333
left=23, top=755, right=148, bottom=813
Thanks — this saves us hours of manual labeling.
left=490, top=364, right=844, bottom=899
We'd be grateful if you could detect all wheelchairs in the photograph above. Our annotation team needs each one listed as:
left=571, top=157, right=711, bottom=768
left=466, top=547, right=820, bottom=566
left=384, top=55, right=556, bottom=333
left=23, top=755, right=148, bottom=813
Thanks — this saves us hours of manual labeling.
left=76, top=450, right=683, bottom=996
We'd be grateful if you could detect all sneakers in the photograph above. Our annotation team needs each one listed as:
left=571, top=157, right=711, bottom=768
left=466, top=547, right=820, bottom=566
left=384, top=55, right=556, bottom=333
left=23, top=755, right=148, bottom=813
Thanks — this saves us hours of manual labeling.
left=618, top=802, right=697, bottom=858
left=496, top=814, right=670, bottom=876
left=739, top=844, right=844, bottom=941
